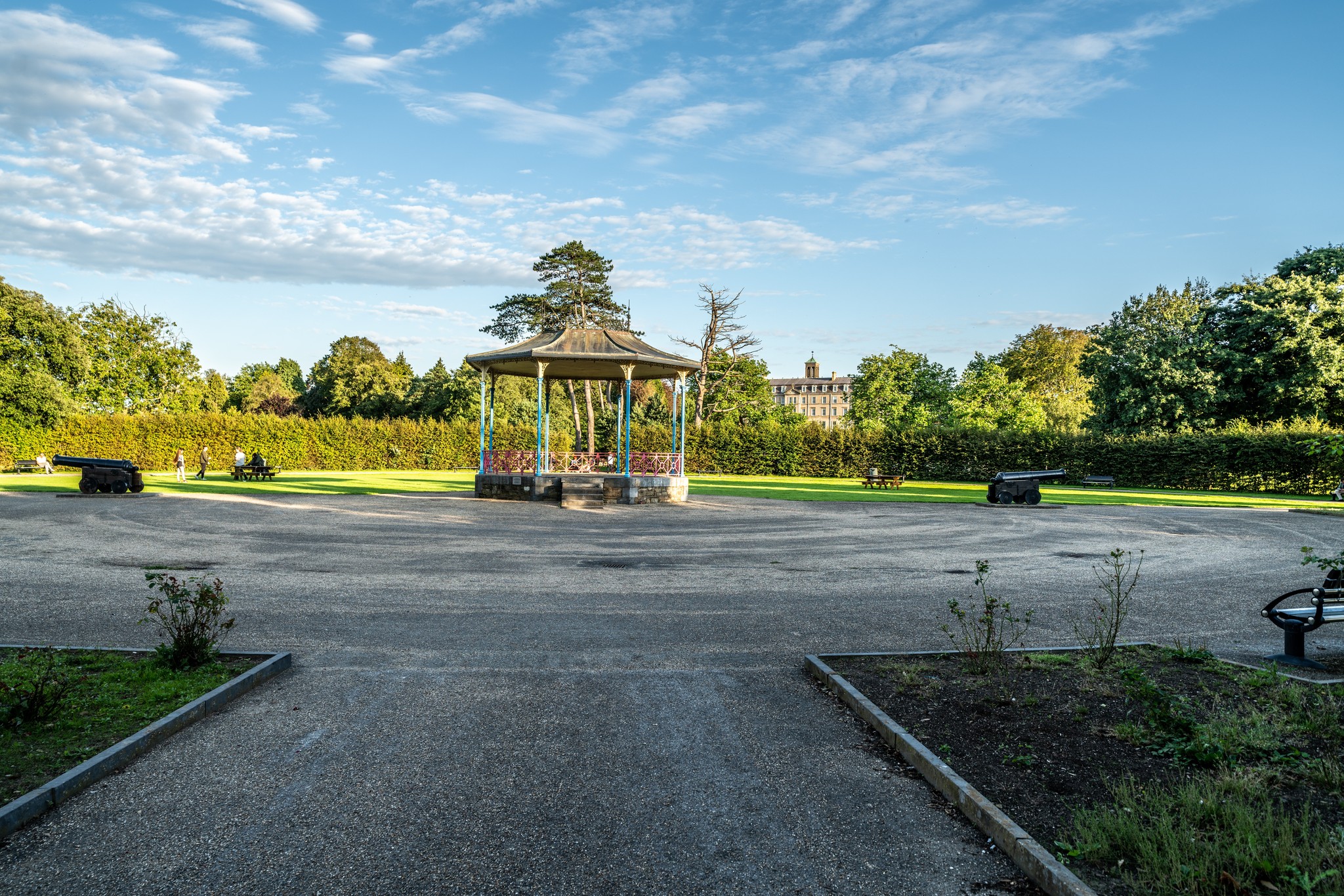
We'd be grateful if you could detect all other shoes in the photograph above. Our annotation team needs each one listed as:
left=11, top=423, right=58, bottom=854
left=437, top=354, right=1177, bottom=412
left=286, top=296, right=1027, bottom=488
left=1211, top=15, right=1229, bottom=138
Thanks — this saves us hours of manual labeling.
left=194, top=475, right=199, bottom=480
left=201, top=478, right=206, bottom=480
left=51, top=470, right=55, bottom=472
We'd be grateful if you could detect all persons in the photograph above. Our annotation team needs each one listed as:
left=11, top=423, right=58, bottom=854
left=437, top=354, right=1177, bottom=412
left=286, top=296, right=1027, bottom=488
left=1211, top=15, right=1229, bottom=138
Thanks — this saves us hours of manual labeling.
left=36, top=453, right=56, bottom=475
left=570, top=456, right=581, bottom=468
left=245, top=453, right=264, bottom=466
left=607, top=452, right=615, bottom=473
left=172, top=448, right=188, bottom=483
left=194, top=445, right=211, bottom=480
left=234, top=447, right=248, bottom=481
left=548, top=450, right=555, bottom=469
left=575, top=459, right=590, bottom=473
left=588, top=456, right=595, bottom=472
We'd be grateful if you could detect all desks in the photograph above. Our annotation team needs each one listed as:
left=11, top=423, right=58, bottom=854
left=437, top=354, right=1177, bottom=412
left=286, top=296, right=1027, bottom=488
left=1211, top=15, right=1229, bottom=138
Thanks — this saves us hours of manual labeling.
left=863, top=475, right=905, bottom=490
left=231, top=465, right=275, bottom=481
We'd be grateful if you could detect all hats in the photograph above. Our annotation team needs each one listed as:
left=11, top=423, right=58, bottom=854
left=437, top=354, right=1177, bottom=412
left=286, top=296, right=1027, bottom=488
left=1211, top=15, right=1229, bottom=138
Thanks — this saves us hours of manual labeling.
left=608, top=452, right=612, bottom=453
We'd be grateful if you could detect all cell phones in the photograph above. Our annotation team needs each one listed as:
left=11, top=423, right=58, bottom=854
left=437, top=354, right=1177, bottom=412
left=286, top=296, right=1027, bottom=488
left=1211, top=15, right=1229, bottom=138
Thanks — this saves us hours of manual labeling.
left=1339, top=489, right=1340, bottom=490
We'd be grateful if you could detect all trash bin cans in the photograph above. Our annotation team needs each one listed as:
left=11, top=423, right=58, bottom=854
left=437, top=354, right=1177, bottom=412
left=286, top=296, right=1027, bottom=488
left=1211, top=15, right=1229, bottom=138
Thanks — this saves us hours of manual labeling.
left=260, top=459, right=266, bottom=476
left=870, top=468, right=878, bottom=481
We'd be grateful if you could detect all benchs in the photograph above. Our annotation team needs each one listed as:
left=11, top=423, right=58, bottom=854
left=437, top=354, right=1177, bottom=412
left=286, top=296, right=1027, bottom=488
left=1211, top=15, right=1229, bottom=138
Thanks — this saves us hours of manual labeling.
left=565, top=468, right=595, bottom=472
left=454, top=465, right=478, bottom=472
left=859, top=480, right=905, bottom=483
left=698, top=469, right=723, bottom=476
left=1081, top=476, right=1115, bottom=489
left=1329, top=485, right=1344, bottom=501
left=1260, top=568, right=1344, bottom=669
left=227, top=472, right=275, bottom=473
left=273, top=464, right=282, bottom=472
left=15, top=460, right=54, bottom=474
